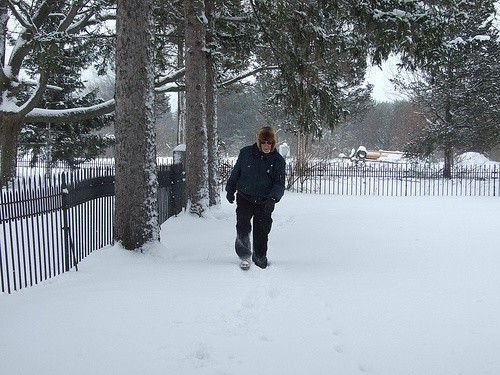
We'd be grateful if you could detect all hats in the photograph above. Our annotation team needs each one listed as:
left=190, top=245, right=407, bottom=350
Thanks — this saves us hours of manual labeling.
left=256, top=127, right=276, bottom=153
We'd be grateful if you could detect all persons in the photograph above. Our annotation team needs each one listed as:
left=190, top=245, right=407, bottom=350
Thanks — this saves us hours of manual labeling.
left=225, top=126, right=287, bottom=270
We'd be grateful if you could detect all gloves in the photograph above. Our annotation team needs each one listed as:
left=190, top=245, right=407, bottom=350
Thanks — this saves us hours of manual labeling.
left=265, top=200, right=275, bottom=213
left=226, top=192, right=235, bottom=204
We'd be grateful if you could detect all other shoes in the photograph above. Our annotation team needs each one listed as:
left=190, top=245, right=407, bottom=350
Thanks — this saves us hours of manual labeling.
left=252, top=255, right=268, bottom=268
left=240, top=258, right=252, bottom=268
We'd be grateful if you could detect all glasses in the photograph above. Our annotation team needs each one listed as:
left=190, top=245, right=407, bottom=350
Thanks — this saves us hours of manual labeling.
left=260, top=141, right=272, bottom=145
